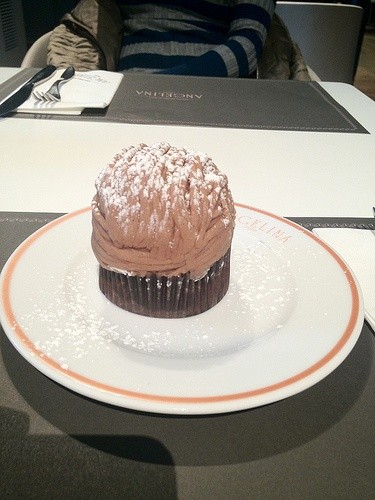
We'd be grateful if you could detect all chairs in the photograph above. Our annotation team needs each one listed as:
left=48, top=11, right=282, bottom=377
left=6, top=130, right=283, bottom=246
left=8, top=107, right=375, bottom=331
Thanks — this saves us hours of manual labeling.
left=21, top=0, right=321, bottom=83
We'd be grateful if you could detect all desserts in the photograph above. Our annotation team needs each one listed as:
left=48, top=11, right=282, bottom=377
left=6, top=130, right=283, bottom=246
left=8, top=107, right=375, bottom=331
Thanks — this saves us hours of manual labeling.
left=90, top=142, right=237, bottom=318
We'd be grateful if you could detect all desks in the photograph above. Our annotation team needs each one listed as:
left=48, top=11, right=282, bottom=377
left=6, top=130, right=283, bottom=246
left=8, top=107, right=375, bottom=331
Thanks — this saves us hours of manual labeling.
left=0, top=66, right=374, bottom=500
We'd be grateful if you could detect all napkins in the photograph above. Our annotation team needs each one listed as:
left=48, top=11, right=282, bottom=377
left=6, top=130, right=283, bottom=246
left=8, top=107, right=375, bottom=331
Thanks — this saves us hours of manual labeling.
left=11, top=66, right=124, bottom=115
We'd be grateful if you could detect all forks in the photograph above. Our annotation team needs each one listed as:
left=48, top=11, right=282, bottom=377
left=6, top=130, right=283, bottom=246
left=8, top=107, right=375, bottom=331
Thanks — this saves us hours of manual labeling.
left=32, top=66, right=75, bottom=103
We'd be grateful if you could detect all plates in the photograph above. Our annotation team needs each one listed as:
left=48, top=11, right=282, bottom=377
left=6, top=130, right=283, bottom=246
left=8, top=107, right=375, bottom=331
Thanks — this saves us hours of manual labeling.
left=0, top=198, right=364, bottom=416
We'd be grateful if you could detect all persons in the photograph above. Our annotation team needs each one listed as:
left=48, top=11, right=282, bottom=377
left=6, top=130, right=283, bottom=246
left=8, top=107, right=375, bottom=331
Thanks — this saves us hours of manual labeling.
left=47, top=0, right=276, bottom=78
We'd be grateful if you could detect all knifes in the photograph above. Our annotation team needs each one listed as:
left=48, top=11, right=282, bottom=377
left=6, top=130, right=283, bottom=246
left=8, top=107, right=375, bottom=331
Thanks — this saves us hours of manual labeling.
left=0, top=65, right=58, bottom=114
